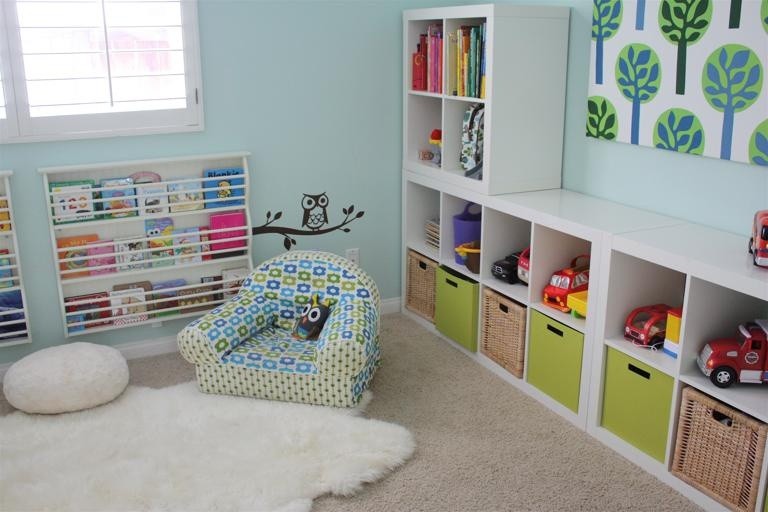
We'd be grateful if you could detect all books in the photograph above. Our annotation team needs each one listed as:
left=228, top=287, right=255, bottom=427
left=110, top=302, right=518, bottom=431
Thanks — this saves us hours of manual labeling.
left=56, top=235, right=145, bottom=280
left=410, top=19, right=485, bottom=100
left=0, top=195, right=28, bottom=341
left=424, top=217, right=440, bottom=250
left=145, top=210, right=247, bottom=269
left=64, top=281, right=155, bottom=332
left=49, top=167, right=246, bottom=226
left=152, top=265, right=251, bottom=318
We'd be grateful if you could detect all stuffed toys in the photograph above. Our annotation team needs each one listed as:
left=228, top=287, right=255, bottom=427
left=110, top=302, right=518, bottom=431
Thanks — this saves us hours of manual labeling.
left=288, top=293, right=338, bottom=344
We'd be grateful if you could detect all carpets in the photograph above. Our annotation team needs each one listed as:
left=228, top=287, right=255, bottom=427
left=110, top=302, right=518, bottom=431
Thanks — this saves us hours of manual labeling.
left=0, top=379, right=416, bottom=511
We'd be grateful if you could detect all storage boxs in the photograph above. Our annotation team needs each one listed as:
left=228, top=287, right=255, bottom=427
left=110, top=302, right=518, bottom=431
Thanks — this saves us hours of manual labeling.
left=527, top=307, right=584, bottom=415
left=602, top=344, right=675, bottom=465
left=435, top=265, right=478, bottom=352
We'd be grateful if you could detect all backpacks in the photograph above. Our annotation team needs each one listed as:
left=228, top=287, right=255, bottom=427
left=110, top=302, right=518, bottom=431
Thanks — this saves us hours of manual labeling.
left=460, top=103, right=484, bottom=171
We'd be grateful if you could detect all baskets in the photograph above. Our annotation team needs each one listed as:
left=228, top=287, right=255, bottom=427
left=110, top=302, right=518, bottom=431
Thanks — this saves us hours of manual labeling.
left=481, top=287, right=527, bottom=379
left=406, top=250, right=438, bottom=324
left=671, top=387, right=768, bottom=512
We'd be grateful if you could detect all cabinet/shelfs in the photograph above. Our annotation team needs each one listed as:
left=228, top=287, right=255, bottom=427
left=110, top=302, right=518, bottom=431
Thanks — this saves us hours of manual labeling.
left=586, top=233, right=766, bottom=512
left=0, top=172, right=32, bottom=346
left=404, top=177, right=602, bottom=436
left=37, top=151, right=252, bottom=337
left=402, top=7, right=568, bottom=195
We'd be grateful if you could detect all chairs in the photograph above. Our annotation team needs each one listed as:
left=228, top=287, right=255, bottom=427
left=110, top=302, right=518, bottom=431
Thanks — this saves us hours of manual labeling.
left=174, top=249, right=382, bottom=408
